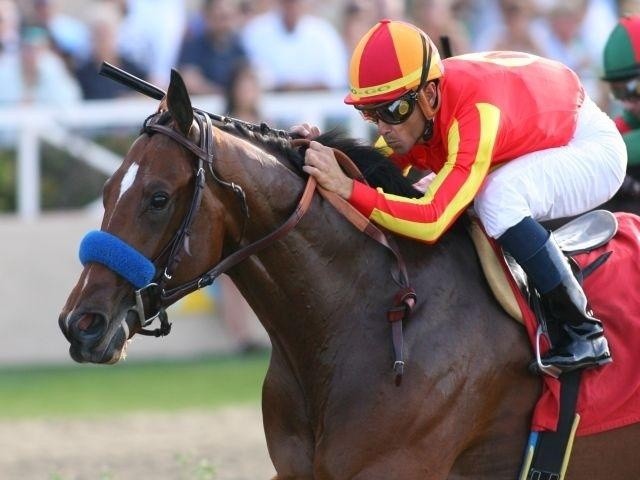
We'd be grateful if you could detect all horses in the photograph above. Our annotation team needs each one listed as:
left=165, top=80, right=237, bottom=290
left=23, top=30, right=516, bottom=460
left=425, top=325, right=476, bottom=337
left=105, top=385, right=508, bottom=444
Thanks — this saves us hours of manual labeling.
left=50, top=65, right=640, bottom=480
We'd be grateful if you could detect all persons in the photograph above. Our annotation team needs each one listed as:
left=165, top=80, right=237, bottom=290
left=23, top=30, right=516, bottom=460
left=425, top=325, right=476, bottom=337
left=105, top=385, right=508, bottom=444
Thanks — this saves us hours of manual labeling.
left=284, top=17, right=631, bottom=376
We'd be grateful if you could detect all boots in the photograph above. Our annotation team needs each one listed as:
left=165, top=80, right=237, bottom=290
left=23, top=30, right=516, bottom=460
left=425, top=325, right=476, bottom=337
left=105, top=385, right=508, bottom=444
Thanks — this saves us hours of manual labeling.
left=518, top=230, right=613, bottom=378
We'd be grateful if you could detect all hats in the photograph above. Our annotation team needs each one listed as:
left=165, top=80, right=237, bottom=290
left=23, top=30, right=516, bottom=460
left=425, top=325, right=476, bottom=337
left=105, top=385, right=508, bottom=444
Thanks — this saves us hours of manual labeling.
left=600, top=14, right=639, bottom=83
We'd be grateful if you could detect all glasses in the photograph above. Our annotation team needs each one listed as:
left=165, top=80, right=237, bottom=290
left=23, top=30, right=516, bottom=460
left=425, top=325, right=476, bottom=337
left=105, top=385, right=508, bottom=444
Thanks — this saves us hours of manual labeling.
left=354, top=89, right=420, bottom=126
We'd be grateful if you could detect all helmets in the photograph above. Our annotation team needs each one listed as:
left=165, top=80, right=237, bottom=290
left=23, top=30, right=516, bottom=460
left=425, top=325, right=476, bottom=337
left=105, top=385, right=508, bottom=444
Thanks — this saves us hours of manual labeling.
left=344, top=18, right=444, bottom=104
